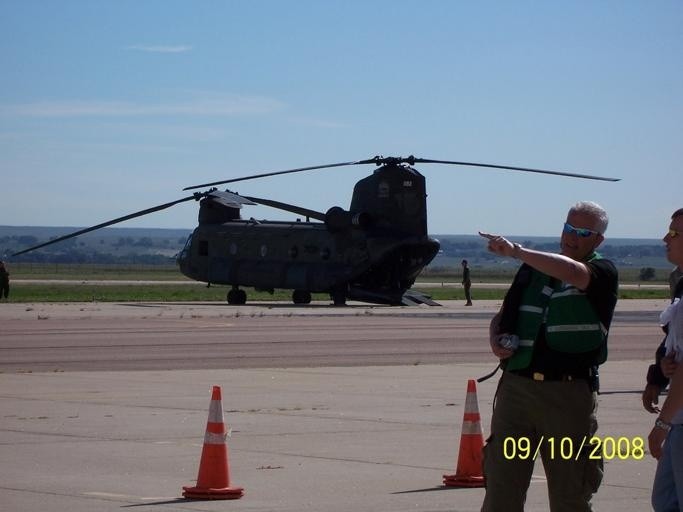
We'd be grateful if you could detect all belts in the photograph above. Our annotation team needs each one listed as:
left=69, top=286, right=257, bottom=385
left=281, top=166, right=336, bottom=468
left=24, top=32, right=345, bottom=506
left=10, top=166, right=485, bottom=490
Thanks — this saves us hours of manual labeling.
left=498, top=362, right=598, bottom=383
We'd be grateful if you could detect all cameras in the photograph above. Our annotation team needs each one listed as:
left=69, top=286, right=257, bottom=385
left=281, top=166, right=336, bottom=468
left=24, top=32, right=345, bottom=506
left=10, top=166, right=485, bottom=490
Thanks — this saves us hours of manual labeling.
left=497, top=334, right=519, bottom=351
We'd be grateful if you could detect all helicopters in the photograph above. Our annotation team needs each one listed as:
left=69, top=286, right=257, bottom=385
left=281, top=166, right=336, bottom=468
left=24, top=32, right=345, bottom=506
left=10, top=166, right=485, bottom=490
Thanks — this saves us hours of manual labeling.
left=12, top=156, right=621, bottom=305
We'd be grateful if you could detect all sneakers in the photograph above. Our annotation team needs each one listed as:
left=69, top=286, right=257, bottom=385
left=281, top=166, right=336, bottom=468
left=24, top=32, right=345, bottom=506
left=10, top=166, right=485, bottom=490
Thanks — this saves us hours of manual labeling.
left=464, top=302, right=472, bottom=306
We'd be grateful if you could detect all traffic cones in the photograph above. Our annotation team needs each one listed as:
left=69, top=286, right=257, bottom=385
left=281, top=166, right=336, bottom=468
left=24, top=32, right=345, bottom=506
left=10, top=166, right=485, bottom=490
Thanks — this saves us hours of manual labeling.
left=181, top=385, right=245, bottom=501
left=441, top=380, right=486, bottom=488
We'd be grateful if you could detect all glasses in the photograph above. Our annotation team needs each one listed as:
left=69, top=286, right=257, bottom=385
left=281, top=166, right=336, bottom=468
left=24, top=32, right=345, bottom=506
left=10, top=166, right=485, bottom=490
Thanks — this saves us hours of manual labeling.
left=666, top=226, right=683, bottom=239
left=560, top=221, right=601, bottom=238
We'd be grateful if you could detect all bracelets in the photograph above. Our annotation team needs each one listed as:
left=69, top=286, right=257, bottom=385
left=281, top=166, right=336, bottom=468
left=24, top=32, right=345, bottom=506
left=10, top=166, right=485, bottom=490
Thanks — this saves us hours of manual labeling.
left=654, top=417, right=672, bottom=433
left=512, top=241, right=523, bottom=258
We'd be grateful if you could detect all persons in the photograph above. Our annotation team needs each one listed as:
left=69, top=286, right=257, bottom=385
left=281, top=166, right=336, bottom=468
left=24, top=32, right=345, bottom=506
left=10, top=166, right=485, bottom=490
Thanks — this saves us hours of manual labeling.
left=475, top=197, right=621, bottom=510
left=461, top=258, right=472, bottom=307
left=642, top=207, right=682, bottom=512
left=647, top=266, right=682, bottom=511
